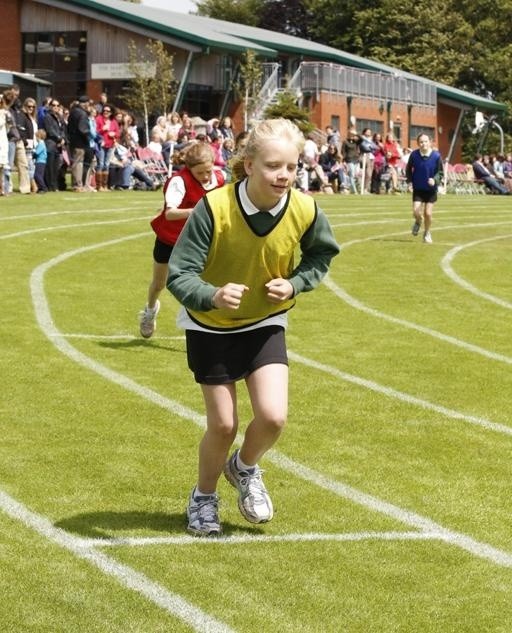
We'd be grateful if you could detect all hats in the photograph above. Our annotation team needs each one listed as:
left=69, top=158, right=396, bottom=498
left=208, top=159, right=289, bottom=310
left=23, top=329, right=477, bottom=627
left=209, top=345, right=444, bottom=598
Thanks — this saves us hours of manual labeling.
left=79, top=95, right=89, bottom=102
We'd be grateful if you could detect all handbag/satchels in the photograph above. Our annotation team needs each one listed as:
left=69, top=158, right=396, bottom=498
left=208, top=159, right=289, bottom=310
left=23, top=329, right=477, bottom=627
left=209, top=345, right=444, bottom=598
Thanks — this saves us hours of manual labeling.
left=6, top=113, right=20, bottom=142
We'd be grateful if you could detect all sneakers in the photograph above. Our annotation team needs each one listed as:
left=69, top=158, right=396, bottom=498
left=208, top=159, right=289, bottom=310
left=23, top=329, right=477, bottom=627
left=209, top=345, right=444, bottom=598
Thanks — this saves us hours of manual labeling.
left=140, top=300, right=160, bottom=338
left=224, top=449, right=273, bottom=524
left=424, top=232, right=432, bottom=243
left=413, top=223, right=419, bottom=236
left=187, top=485, right=221, bottom=535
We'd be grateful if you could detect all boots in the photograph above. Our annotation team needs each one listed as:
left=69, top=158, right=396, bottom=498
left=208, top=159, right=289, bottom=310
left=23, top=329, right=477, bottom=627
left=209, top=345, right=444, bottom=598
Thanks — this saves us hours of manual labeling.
left=95, top=172, right=111, bottom=192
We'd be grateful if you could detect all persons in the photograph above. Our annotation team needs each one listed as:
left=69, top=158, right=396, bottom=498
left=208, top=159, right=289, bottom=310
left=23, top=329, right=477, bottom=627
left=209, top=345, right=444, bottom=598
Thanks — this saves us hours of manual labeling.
left=166, top=114, right=342, bottom=538
left=137, top=141, right=226, bottom=343
left=403, top=134, right=446, bottom=246
left=0, top=82, right=413, bottom=195
left=472, top=152, right=511, bottom=196
